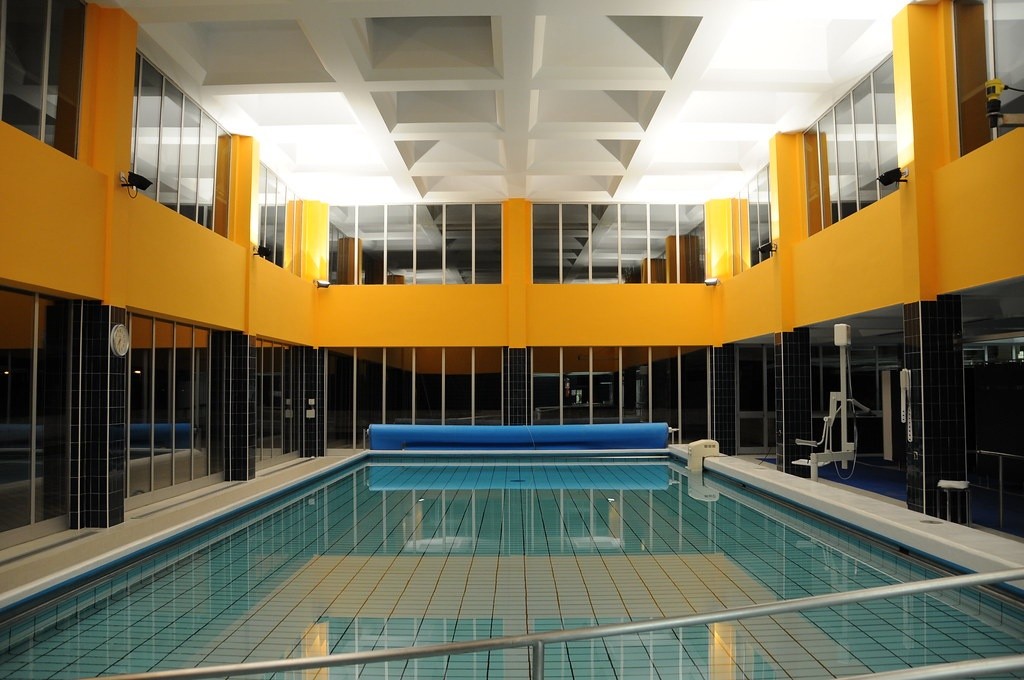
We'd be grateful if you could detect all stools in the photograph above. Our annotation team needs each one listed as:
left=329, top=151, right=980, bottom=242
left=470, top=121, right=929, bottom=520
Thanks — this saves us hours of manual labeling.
left=937, top=487, right=971, bottom=526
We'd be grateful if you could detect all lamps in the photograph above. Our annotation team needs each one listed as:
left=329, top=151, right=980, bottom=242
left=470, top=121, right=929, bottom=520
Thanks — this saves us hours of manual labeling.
left=313, top=279, right=330, bottom=288
left=121, top=171, right=153, bottom=199
left=877, top=167, right=908, bottom=186
left=704, top=278, right=720, bottom=286
left=253, top=245, right=272, bottom=257
left=757, top=241, right=777, bottom=254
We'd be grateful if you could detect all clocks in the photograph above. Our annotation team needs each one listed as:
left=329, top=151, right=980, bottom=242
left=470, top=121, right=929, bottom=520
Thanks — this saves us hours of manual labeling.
left=110, top=323, right=129, bottom=357
